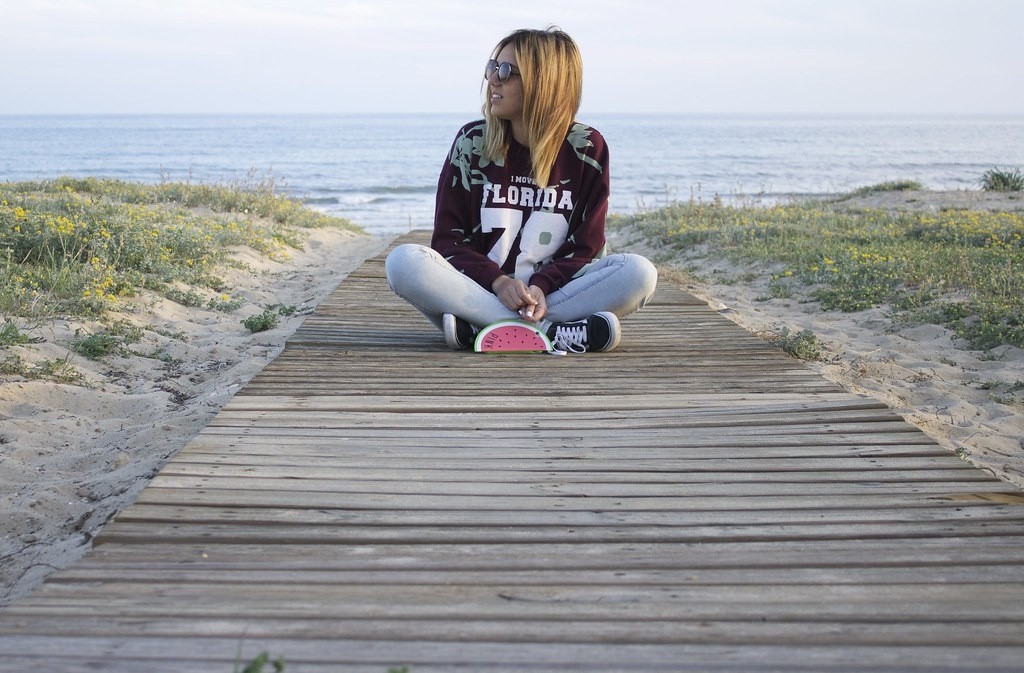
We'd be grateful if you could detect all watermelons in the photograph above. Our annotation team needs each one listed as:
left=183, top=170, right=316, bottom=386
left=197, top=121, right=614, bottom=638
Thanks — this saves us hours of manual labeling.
left=474, top=320, right=553, bottom=352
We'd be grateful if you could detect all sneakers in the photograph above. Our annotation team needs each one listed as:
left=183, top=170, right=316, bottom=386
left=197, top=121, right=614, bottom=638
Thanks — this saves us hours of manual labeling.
left=549, top=311, right=622, bottom=356
left=442, top=313, right=485, bottom=350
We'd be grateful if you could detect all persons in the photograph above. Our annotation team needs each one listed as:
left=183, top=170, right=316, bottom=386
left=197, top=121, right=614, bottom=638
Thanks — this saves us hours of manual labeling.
left=385, top=25, right=658, bottom=356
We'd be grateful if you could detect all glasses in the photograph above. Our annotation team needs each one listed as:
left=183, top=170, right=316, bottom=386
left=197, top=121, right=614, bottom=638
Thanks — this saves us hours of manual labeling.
left=484, top=59, right=520, bottom=84
